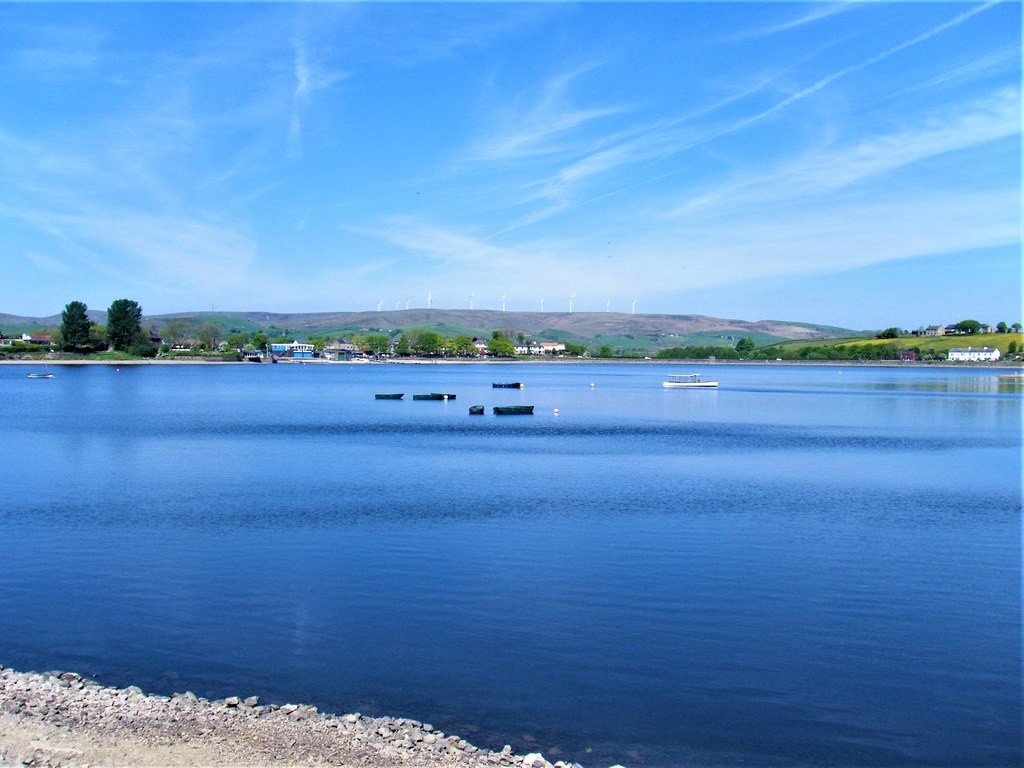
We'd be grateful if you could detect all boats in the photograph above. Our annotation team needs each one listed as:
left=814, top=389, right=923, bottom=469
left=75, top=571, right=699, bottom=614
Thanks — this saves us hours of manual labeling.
left=413, top=393, right=456, bottom=400
left=662, top=374, right=718, bottom=388
left=469, top=405, right=484, bottom=414
left=493, top=405, right=534, bottom=413
left=28, top=373, right=54, bottom=378
left=376, top=393, right=405, bottom=398
left=493, top=383, right=522, bottom=388
